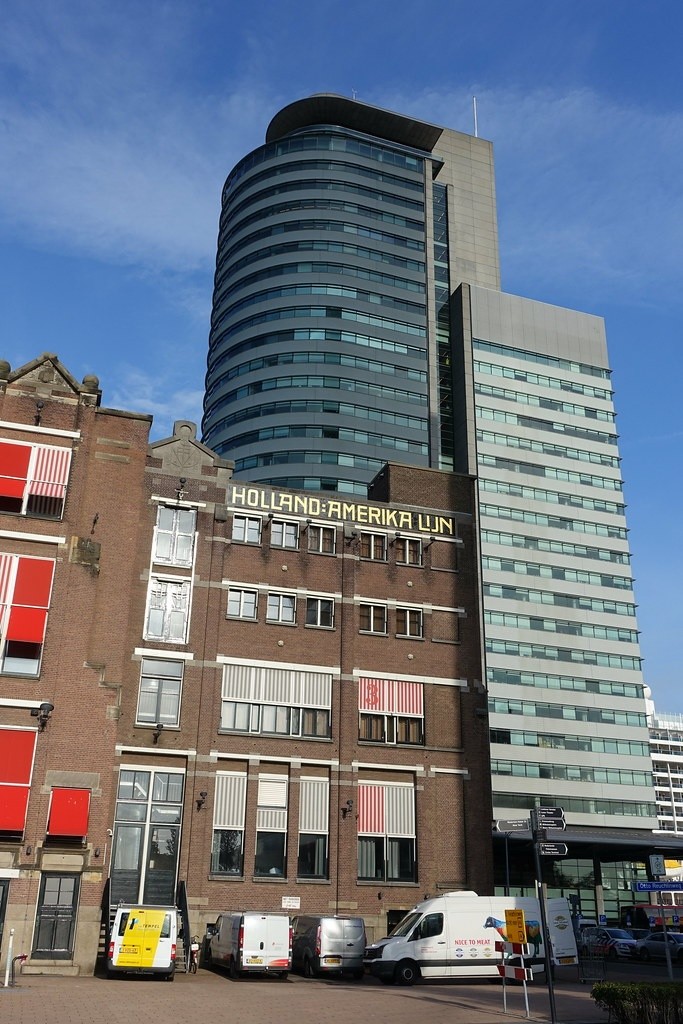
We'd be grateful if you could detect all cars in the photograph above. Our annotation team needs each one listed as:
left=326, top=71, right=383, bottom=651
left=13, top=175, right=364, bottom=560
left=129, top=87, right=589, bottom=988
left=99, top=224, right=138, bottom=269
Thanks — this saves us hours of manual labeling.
left=636, top=931, right=683, bottom=964
left=581, top=927, right=637, bottom=963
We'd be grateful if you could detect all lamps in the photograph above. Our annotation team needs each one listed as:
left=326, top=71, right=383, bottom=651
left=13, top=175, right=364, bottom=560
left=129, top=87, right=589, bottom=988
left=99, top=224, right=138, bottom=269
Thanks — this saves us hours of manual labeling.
left=152, top=723, right=164, bottom=744
left=345, top=532, right=358, bottom=545
left=264, top=513, right=274, bottom=529
left=424, top=537, right=436, bottom=550
left=37, top=701, right=55, bottom=733
left=173, top=478, right=187, bottom=500
left=389, top=532, right=401, bottom=547
left=195, top=791, right=207, bottom=812
left=300, top=519, right=313, bottom=535
left=33, top=401, right=44, bottom=425
left=341, top=800, right=353, bottom=818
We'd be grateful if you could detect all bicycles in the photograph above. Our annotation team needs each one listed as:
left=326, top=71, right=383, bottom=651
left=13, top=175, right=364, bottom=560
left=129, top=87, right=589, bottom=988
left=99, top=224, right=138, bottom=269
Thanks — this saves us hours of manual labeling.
left=184, top=934, right=199, bottom=973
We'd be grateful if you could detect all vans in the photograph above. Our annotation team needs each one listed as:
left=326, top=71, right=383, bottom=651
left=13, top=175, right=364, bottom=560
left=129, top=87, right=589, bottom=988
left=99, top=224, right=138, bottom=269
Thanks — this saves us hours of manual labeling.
left=290, top=914, right=367, bottom=976
left=107, top=903, right=179, bottom=982
left=201, top=908, right=293, bottom=980
left=364, top=890, right=579, bottom=985
